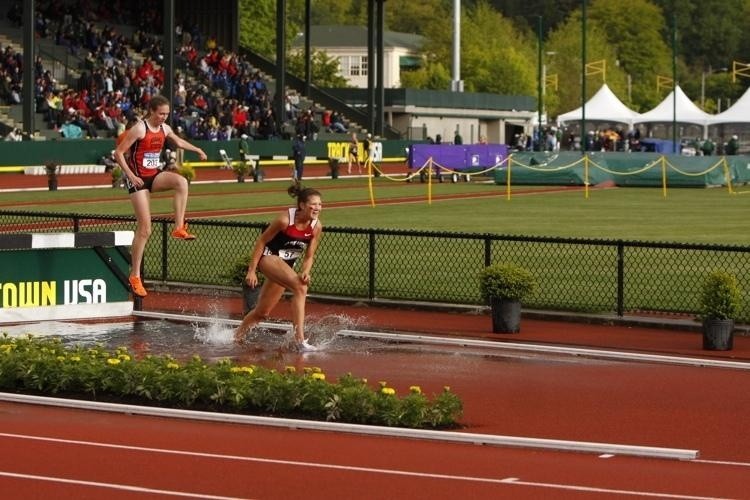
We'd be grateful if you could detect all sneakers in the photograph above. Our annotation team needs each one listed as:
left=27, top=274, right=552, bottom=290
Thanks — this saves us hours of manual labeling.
left=295, top=339, right=319, bottom=352
left=172, top=226, right=196, bottom=241
left=129, top=271, right=147, bottom=297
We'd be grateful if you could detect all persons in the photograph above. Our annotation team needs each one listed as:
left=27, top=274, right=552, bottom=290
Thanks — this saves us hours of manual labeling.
left=515, top=124, right=644, bottom=152
left=0, top=10, right=374, bottom=179
left=114, top=97, right=208, bottom=297
left=228, top=182, right=323, bottom=351
left=688, top=134, right=740, bottom=155
left=479, top=135, right=488, bottom=145
left=453, top=130, right=463, bottom=145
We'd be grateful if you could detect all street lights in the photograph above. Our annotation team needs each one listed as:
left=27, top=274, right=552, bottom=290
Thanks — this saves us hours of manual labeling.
left=701, top=68, right=728, bottom=108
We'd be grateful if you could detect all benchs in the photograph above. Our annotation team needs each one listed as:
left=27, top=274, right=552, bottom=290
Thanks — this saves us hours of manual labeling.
left=1, top=0, right=116, bottom=141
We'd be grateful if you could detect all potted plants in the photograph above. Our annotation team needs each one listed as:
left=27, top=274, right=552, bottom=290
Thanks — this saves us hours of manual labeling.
left=478, top=264, right=542, bottom=334
left=698, top=270, right=743, bottom=351
left=177, top=161, right=195, bottom=186
left=373, top=164, right=381, bottom=177
left=111, top=166, right=122, bottom=188
left=234, top=163, right=249, bottom=182
